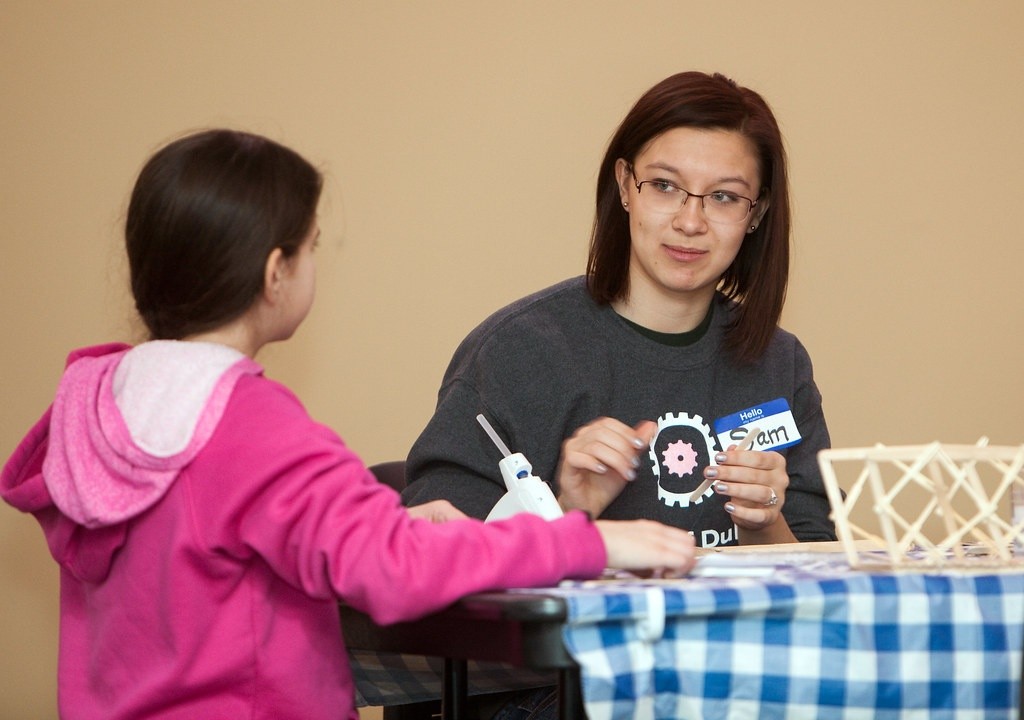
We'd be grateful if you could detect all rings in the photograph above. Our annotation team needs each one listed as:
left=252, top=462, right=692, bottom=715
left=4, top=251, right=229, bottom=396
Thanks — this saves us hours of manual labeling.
left=764, top=488, right=777, bottom=506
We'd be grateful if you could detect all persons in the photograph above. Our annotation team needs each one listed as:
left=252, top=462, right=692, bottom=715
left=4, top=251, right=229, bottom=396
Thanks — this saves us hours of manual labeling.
left=0, top=131, right=696, bottom=720
left=399, top=71, right=844, bottom=547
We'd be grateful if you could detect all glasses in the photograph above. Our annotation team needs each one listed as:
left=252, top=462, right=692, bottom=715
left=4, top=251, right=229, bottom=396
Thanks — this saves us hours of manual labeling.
left=626, top=162, right=763, bottom=225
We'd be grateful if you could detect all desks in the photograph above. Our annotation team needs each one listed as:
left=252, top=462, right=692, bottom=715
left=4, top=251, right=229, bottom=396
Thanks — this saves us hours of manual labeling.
left=342, top=540, right=1024, bottom=720
